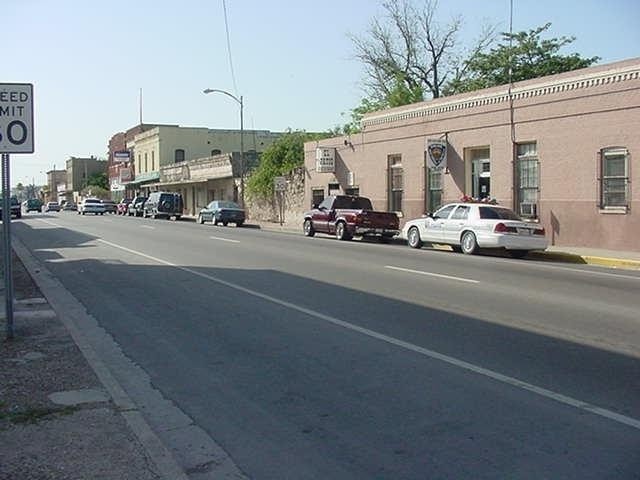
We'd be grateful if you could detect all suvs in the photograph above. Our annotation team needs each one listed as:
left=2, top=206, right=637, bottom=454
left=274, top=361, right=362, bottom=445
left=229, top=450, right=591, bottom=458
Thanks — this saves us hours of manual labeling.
left=0, top=198, right=21, bottom=219
left=24, top=199, right=42, bottom=213
left=143, top=191, right=184, bottom=221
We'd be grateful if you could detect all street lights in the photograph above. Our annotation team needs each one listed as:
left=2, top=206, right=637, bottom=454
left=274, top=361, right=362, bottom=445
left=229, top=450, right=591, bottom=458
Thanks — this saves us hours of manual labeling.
left=203, top=88, right=244, bottom=208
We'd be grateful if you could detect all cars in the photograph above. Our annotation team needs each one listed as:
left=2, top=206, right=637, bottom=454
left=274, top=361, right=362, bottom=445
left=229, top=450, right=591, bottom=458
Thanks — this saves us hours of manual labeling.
left=44, top=202, right=60, bottom=212
left=60, top=196, right=148, bottom=216
left=197, top=199, right=246, bottom=226
left=400, top=197, right=548, bottom=258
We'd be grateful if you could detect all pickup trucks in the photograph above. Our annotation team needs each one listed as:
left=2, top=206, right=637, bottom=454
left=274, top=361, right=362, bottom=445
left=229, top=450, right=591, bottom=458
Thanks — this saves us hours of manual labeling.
left=302, top=194, right=400, bottom=242
